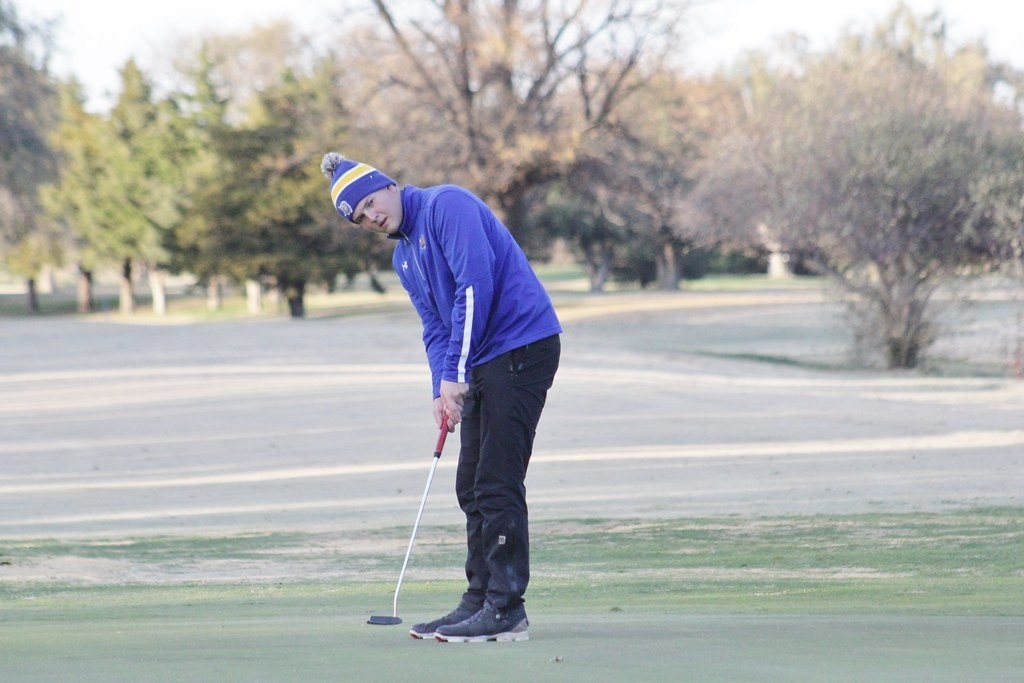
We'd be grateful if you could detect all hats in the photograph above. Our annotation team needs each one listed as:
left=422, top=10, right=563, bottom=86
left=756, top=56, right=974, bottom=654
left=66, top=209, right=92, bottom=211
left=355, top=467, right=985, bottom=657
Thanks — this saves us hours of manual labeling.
left=322, top=151, right=396, bottom=224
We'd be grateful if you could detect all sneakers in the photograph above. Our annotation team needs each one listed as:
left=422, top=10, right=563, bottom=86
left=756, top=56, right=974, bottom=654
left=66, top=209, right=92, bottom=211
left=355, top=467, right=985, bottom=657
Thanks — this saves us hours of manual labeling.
left=435, top=599, right=530, bottom=642
left=409, top=592, right=486, bottom=639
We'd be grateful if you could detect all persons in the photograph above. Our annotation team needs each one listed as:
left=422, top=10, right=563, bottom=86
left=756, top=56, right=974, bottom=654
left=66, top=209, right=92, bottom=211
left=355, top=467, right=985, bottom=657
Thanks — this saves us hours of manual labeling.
left=319, top=151, right=561, bottom=644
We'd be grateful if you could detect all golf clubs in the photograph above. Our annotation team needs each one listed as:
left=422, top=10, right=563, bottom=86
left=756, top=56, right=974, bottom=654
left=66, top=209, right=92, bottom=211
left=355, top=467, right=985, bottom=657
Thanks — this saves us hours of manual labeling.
left=367, top=407, right=452, bottom=626
